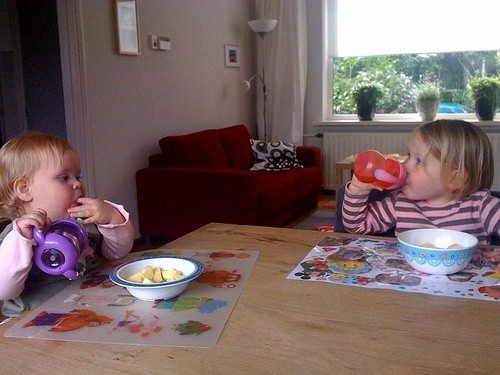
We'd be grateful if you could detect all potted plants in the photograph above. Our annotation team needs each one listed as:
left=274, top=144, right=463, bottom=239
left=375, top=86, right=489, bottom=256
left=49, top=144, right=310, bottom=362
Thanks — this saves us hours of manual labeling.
left=414, top=81, right=439, bottom=122
left=349, top=82, right=390, bottom=120
left=466, top=74, right=500, bottom=122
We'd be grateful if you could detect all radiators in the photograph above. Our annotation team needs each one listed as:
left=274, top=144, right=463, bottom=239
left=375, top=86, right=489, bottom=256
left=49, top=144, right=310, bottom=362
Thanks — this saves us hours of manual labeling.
left=326, top=131, right=500, bottom=194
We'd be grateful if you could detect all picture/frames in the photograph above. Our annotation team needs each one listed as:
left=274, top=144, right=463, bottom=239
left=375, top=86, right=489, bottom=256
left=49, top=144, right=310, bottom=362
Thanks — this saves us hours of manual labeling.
left=114, top=0, right=142, bottom=55
left=224, top=42, right=242, bottom=69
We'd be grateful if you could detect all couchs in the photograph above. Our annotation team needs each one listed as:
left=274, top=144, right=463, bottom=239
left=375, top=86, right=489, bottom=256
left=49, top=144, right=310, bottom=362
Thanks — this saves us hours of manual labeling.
left=136, top=144, right=324, bottom=247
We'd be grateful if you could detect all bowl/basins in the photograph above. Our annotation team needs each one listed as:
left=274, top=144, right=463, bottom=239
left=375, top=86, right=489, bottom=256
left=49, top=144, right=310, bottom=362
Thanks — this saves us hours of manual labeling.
left=397, top=228, right=478, bottom=274
left=109, top=255, right=204, bottom=302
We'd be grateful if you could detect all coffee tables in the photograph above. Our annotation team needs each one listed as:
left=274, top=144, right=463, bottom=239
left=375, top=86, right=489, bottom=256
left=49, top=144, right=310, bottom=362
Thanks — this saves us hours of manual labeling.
left=334, top=154, right=407, bottom=203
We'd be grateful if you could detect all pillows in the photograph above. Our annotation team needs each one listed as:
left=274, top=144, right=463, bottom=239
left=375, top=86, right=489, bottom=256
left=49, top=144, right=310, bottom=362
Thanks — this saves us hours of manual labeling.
left=246, top=140, right=303, bottom=172
left=220, top=123, right=253, bottom=168
left=159, top=129, right=233, bottom=167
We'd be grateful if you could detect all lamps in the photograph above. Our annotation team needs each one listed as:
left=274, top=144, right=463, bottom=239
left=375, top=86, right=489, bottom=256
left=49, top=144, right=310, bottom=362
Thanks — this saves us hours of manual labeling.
left=240, top=18, right=277, bottom=142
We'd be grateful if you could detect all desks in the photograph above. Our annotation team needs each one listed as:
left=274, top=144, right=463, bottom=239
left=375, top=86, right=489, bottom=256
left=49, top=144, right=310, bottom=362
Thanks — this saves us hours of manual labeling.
left=0, top=222, right=498, bottom=375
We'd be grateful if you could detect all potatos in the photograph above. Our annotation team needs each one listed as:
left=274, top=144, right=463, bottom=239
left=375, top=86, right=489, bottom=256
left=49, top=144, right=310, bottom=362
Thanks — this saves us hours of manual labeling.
left=417, top=242, right=463, bottom=250
left=127, top=266, right=185, bottom=283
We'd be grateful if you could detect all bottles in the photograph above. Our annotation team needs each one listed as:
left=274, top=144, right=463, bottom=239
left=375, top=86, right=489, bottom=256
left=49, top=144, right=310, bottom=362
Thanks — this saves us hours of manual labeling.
left=32, top=217, right=90, bottom=280
left=353, top=149, right=408, bottom=190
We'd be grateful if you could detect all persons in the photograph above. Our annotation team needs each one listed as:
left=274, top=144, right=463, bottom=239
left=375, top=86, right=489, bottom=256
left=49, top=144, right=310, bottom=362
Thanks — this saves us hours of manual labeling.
left=342, top=119, right=500, bottom=245
left=0, top=132, right=134, bottom=326
